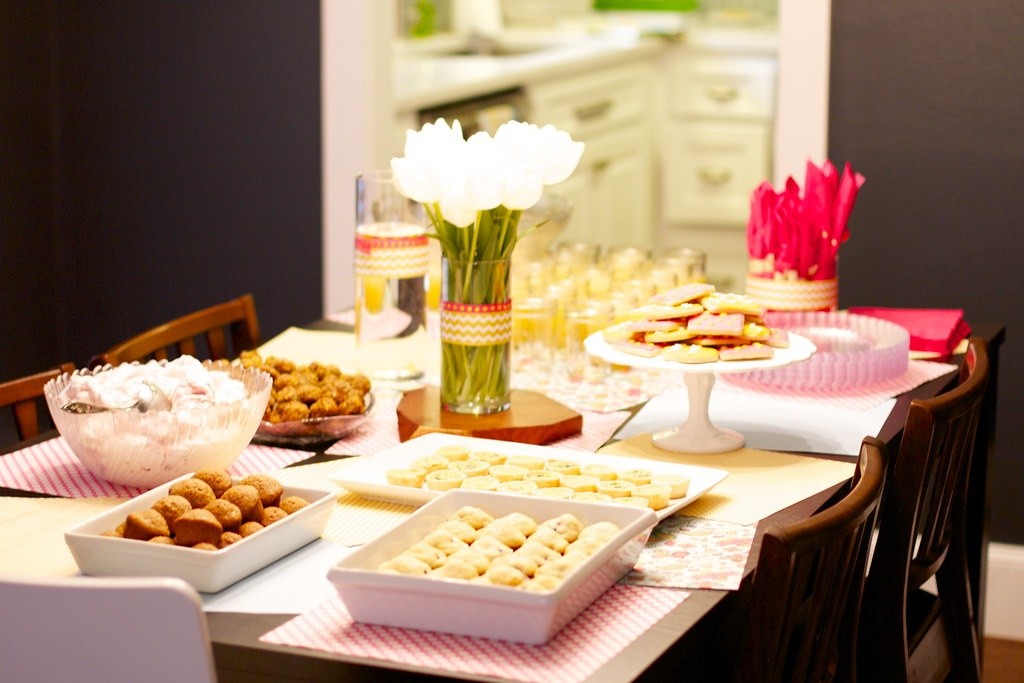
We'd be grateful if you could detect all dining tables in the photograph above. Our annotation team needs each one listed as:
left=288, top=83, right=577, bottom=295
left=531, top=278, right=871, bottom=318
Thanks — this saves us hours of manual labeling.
left=0, top=309, right=1005, bottom=683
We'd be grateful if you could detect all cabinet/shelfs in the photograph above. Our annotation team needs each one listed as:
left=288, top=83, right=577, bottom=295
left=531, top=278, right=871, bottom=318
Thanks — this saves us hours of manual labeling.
left=527, top=62, right=655, bottom=255
left=660, top=50, right=776, bottom=229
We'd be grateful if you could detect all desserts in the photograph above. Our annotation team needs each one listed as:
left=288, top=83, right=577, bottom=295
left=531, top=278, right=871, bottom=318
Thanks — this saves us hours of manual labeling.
left=389, top=445, right=690, bottom=510
left=98, top=469, right=310, bottom=550
left=379, top=505, right=621, bottom=592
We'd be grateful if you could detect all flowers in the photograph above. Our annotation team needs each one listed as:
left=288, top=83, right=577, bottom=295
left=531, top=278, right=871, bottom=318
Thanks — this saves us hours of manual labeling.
left=388, top=117, right=586, bottom=260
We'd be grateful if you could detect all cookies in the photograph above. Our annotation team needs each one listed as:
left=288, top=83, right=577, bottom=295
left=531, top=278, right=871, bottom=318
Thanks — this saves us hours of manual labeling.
left=602, top=281, right=789, bottom=362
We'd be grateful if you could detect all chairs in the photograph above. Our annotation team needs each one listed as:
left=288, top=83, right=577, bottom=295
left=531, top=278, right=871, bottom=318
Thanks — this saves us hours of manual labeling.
left=665, top=435, right=889, bottom=683
left=90, top=294, right=260, bottom=367
left=859, top=335, right=989, bottom=683
left=0, top=574, right=216, bottom=683
left=0, top=362, right=75, bottom=441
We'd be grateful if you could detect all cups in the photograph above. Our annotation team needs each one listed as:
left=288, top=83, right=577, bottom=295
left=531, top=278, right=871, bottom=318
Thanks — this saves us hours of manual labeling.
left=354, top=171, right=433, bottom=380
left=499, top=238, right=711, bottom=411
left=743, top=272, right=841, bottom=314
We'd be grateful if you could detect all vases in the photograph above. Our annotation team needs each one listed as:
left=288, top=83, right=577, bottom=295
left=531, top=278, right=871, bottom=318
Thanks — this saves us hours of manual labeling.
left=441, top=257, right=514, bottom=418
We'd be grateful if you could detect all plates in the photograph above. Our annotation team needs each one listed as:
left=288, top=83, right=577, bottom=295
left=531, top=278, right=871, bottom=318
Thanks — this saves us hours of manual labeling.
left=325, top=488, right=657, bottom=645
left=65, top=469, right=334, bottom=595
left=331, top=430, right=730, bottom=531
left=255, top=388, right=374, bottom=444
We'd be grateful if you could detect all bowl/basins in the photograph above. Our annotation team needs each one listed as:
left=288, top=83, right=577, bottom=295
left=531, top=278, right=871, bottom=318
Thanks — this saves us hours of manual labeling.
left=44, top=358, right=274, bottom=491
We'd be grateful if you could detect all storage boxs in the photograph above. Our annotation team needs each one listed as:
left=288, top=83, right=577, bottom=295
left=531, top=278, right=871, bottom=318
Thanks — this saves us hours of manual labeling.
left=326, top=488, right=658, bottom=646
left=63, top=472, right=336, bottom=594
